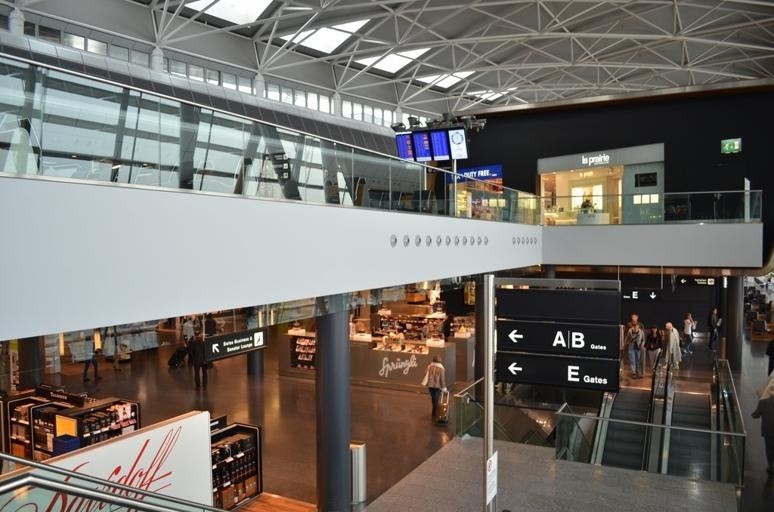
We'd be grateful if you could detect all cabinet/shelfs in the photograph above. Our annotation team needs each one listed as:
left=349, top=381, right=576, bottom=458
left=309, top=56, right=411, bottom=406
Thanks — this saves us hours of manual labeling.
left=1, top=384, right=140, bottom=464
left=211, top=415, right=263, bottom=510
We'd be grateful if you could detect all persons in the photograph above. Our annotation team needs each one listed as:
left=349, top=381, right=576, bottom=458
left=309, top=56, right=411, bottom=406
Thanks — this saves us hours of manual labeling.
left=708, top=308, right=722, bottom=352
left=624, top=312, right=698, bottom=380
left=421, top=356, right=448, bottom=420
left=82, top=336, right=102, bottom=382
left=751, top=338, right=774, bottom=478
left=182, top=313, right=217, bottom=391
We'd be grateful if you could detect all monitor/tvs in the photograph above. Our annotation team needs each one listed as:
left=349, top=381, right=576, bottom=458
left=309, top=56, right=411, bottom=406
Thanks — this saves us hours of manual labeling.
left=412, top=131, right=432, bottom=163
left=720, top=136, right=744, bottom=160
left=448, top=129, right=469, bottom=160
left=430, top=131, right=450, bottom=162
left=396, top=134, right=415, bottom=164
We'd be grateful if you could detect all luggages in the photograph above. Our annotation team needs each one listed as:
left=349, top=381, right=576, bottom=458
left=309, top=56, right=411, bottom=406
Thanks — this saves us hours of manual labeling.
left=435, top=391, right=450, bottom=426
left=167, top=346, right=189, bottom=367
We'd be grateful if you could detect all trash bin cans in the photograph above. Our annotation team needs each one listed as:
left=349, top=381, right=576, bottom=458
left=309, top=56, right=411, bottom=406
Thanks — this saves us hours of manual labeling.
left=52, top=434, right=78, bottom=456
left=350, top=440, right=367, bottom=505
left=247, top=349, right=264, bottom=377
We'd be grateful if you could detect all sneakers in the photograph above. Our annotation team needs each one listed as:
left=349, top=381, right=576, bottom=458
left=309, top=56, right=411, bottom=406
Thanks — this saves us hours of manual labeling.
left=83, top=375, right=103, bottom=381
left=195, top=385, right=209, bottom=393
left=631, top=364, right=681, bottom=379
